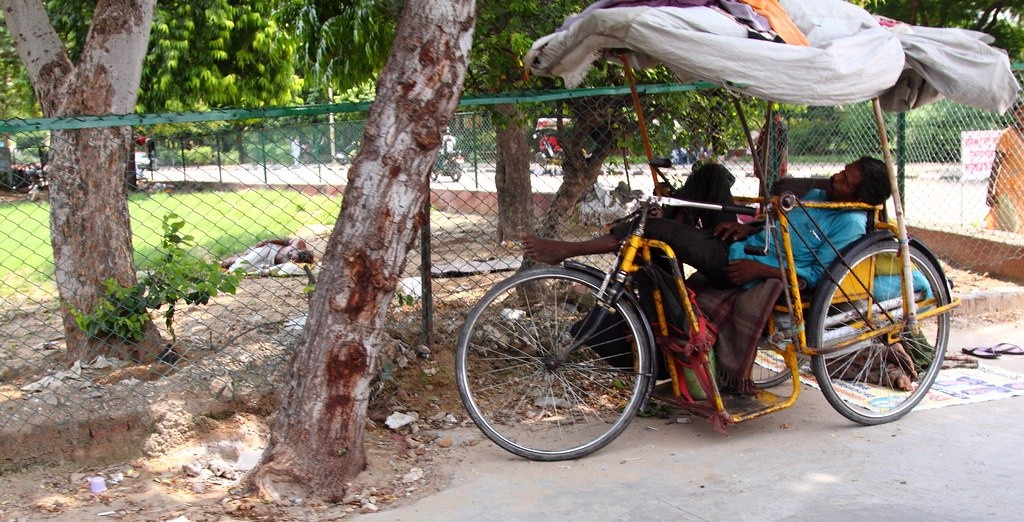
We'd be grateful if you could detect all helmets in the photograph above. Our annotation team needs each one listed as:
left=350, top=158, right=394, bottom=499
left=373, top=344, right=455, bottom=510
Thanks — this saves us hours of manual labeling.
left=445, top=127, right=451, bottom=134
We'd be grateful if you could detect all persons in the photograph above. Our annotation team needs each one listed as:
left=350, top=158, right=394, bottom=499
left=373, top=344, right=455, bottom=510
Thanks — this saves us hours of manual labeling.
left=539, top=132, right=564, bottom=172
left=754, top=104, right=789, bottom=209
left=520, top=155, right=891, bottom=288
left=215, top=236, right=316, bottom=271
left=986, top=97, right=1024, bottom=235
left=431, top=127, right=457, bottom=179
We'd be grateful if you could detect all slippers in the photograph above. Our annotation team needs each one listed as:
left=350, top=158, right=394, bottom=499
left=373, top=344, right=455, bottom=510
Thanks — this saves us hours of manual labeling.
left=961, top=346, right=996, bottom=359
left=986, top=343, right=1024, bottom=354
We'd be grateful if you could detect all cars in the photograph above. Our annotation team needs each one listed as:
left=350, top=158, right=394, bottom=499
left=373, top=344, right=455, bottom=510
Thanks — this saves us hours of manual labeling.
left=134, top=151, right=155, bottom=171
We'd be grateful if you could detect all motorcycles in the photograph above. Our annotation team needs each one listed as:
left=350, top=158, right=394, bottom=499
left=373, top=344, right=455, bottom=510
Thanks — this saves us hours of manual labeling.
left=430, top=150, right=465, bottom=182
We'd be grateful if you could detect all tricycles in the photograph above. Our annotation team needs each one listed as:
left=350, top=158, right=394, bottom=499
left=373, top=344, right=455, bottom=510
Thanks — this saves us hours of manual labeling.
left=455, top=0, right=960, bottom=463
left=533, top=116, right=586, bottom=176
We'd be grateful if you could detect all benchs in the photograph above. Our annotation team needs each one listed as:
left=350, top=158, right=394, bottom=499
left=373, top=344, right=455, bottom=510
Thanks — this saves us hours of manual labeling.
left=767, top=177, right=883, bottom=303
